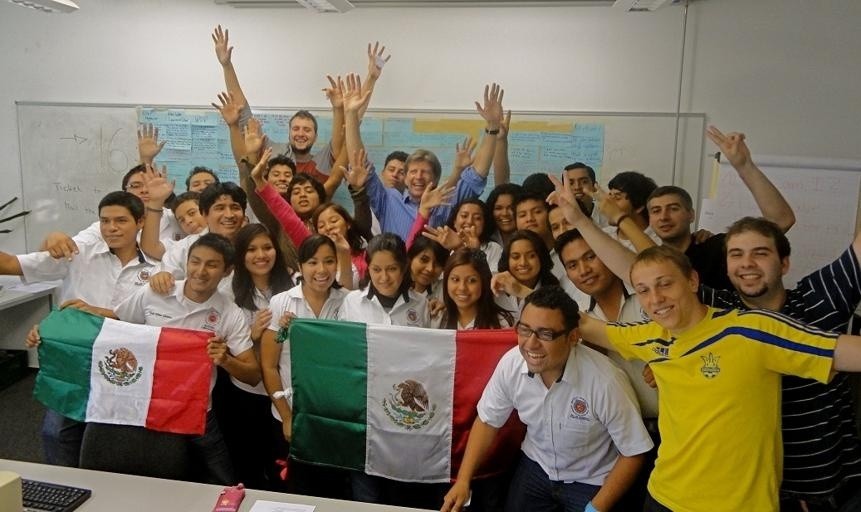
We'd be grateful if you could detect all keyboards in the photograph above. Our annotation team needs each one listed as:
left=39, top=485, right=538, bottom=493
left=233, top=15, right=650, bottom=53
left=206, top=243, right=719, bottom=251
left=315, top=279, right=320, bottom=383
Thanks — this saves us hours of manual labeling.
left=22, top=478, right=92, bottom=512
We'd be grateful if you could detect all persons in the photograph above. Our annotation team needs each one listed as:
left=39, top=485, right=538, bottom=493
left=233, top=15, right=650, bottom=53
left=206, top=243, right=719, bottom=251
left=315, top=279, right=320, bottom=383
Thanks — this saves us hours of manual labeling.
left=1, top=24, right=859, bottom=512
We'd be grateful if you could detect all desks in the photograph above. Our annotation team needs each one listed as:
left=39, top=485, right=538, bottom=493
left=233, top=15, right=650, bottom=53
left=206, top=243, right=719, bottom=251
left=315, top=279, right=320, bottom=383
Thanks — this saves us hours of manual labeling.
left=0, top=459, right=439, bottom=512
left=0, top=275, right=57, bottom=350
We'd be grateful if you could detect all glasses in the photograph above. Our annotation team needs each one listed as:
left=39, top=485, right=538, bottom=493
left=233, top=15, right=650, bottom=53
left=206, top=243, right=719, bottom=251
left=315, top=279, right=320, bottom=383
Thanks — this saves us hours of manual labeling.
left=514, top=320, right=575, bottom=342
